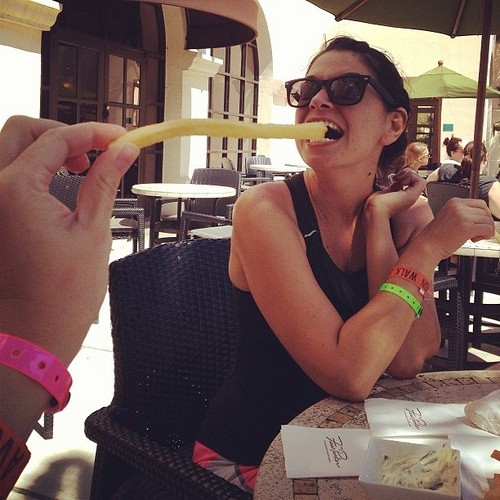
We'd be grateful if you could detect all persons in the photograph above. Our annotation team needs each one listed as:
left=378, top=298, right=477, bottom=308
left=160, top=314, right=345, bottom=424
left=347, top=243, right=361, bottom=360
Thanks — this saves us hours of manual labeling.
left=400, top=136, right=500, bottom=220
left=0, top=118, right=142, bottom=500
left=191, top=35, right=495, bottom=492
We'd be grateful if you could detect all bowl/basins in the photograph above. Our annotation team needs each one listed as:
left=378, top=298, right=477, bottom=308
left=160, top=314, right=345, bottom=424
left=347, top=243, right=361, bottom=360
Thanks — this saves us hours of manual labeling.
left=358, top=437, right=461, bottom=500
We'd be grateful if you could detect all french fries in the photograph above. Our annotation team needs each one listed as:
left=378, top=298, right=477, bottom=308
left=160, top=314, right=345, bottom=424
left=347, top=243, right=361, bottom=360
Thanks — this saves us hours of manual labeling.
left=482, top=449, right=500, bottom=500
left=106, top=120, right=328, bottom=149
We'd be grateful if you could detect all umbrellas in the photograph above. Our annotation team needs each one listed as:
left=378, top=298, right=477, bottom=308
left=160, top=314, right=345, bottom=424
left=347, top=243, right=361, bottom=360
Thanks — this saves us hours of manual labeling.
left=309, top=0, right=500, bottom=200
left=402, top=60, right=500, bottom=152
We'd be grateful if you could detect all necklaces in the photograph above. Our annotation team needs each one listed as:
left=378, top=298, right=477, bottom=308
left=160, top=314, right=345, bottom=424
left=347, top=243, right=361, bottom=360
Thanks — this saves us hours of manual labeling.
left=306, top=168, right=362, bottom=271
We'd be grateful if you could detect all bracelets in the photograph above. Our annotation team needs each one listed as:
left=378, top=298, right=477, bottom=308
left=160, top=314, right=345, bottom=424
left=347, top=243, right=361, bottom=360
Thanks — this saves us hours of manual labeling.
left=0, top=334, right=72, bottom=414
left=380, top=282, right=424, bottom=319
left=0, top=415, right=31, bottom=500
left=389, top=265, right=430, bottom=300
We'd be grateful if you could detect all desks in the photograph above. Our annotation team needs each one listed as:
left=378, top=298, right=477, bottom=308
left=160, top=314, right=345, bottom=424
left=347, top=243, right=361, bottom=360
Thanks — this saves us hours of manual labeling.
left=254, top=370, right=500, bottom=500
left=131, top=184, right=237, bottom=237
left=249, top=165, right=305, bottom=179
left=453, top=240, right=500, bottom=353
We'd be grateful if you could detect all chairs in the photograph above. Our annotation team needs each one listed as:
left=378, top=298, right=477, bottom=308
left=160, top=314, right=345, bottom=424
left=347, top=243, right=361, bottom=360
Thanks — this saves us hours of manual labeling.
left=425, top=181, right=470, bottom=216
left=189, top=225, right=233, bottom=239
left=245, top=156, right=272, bottom=177
left=150, top=168, right=240, bottom=247
left=49, top=175, right=145, bottom=253
left=84, top=237, right=252, bottom=500
left=433, top=258, right=500, bottom=370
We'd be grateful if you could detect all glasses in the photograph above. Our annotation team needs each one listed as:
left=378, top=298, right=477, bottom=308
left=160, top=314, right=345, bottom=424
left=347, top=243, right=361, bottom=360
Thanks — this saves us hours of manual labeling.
left=286, top=73, right=398, bottom=108
left=454, top=149, right=465, bottom=153
left=420, top=153, right=432, bottom=159
